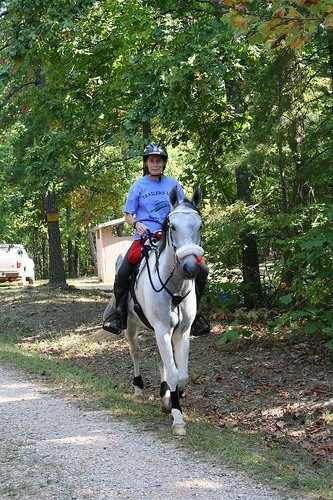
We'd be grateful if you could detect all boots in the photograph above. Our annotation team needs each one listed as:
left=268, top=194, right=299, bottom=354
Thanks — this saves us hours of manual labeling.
left=109, top=275, right=129, bottom=329
left=192, top=279, right=209, bottom=333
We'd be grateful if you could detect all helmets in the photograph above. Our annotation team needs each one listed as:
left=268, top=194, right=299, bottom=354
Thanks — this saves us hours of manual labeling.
left=143, top=144, right=168, bottom=157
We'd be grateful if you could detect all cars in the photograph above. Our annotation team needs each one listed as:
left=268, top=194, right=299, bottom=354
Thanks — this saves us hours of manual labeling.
left=0, top=243, right=35, bottom=286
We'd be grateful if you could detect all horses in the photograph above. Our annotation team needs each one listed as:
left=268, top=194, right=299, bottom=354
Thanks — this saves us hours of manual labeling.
left=90, top=186, right=204, bottom=436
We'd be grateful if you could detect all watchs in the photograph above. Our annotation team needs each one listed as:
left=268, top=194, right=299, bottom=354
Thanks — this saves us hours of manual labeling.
left=132, top=221, right=139, bottom=228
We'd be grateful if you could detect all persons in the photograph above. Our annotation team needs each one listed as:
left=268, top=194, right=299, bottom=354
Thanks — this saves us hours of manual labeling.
left=107, top=142, right=210, bottom=336
